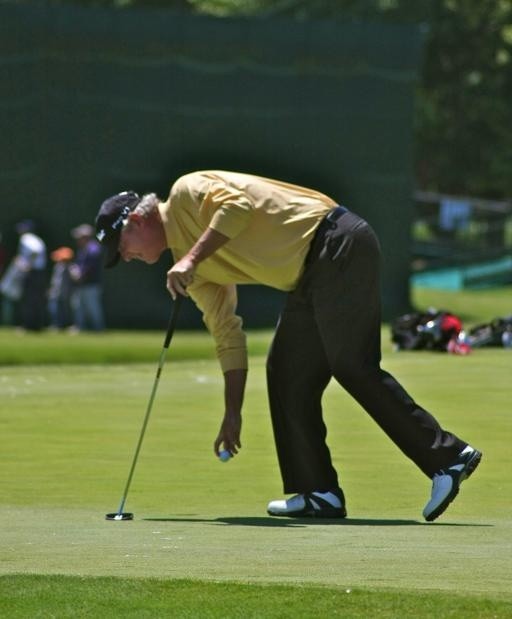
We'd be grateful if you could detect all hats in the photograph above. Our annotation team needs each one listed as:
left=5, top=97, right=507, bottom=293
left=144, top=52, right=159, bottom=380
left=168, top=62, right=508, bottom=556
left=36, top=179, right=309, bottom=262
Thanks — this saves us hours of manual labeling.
left=92, top=190, right=142, bottom=270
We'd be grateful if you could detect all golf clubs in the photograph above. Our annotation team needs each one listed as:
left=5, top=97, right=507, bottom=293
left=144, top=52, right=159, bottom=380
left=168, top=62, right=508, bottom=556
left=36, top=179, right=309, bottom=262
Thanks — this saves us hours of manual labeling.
left=105, top=282, right=187, bottom=521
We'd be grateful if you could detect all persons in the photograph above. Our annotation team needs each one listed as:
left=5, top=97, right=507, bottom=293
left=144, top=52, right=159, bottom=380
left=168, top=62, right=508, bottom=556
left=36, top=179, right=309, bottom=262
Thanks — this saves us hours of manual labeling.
left=46, top=244, right=72, bottom=332
left=93, top=167, right=482, bottom=523
left=67, top=220, right=105, bottom=334
left=14, top=217, right=48, bottom=334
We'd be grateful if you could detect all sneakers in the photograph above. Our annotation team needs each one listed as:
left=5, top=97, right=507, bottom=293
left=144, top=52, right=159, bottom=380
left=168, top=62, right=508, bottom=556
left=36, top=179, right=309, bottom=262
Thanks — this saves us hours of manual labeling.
left=265, top=486, right=349, bottom=519
left=421, top=442, right=484, bottom=523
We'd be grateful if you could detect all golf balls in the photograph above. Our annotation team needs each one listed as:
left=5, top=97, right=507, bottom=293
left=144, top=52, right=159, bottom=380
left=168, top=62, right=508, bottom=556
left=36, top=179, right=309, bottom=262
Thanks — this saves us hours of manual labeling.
left=220, top=450, right=231, bottom=461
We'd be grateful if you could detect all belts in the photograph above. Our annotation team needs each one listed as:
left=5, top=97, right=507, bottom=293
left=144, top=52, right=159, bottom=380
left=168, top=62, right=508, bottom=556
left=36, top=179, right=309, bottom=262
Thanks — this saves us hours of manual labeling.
left=302, top=206, right=351, bottom=276
